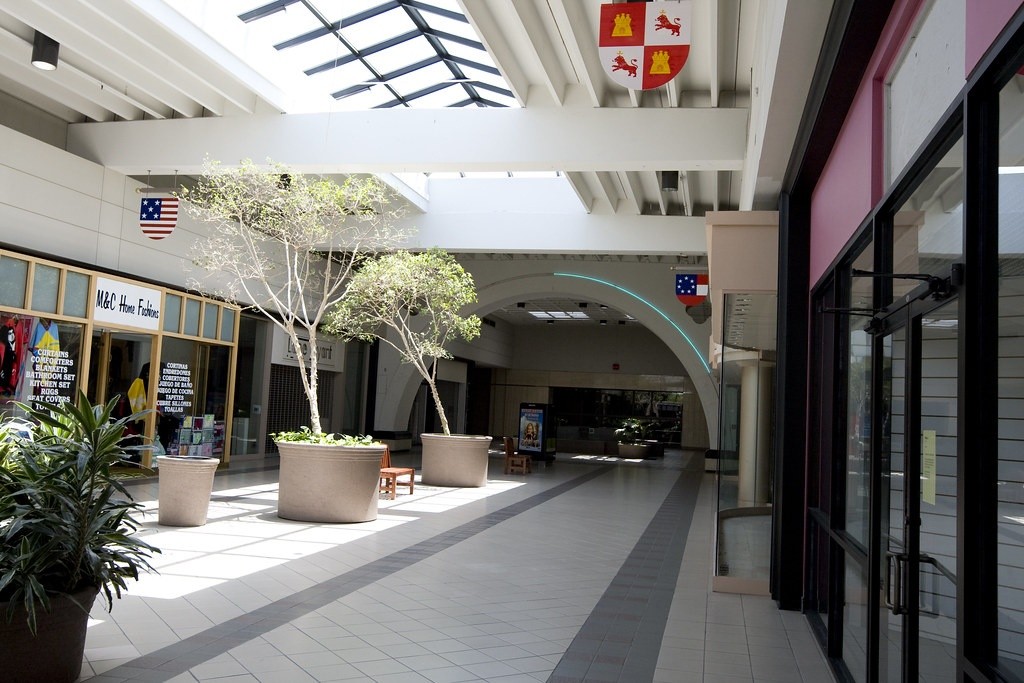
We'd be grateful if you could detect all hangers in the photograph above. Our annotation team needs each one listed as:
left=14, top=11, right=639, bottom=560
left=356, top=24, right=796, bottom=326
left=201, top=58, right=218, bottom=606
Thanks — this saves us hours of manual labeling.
left=11, top=314, right=19, bottom=321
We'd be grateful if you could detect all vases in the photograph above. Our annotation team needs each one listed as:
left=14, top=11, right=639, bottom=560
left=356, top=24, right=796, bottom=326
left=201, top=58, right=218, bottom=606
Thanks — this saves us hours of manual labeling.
left=156, top=454, right=220, bottom=527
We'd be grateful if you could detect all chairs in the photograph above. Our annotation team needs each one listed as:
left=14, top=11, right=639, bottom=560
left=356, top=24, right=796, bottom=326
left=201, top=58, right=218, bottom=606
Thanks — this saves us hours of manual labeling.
left=379, top=444, right=414, bottom=499
left=503, top=437, right=534, bottom=475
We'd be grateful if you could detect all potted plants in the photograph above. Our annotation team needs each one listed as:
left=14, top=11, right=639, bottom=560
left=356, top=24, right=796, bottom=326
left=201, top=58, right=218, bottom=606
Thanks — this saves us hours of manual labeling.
left=616, top=418, right=646, bottom=459
left=173, top=150, right=493, bottom=523
left=0, top=387, right=164, bottom=683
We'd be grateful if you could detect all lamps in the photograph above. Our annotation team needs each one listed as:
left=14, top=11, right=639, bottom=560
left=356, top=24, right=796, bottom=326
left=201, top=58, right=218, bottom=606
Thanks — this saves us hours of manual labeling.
left=662, top=170, right=679, bottom=192
left=31, top=28, right=59, bottom=72
left=517, top=301, right=626, bottom=327
left=276, top=174, right=292, bottom=193
